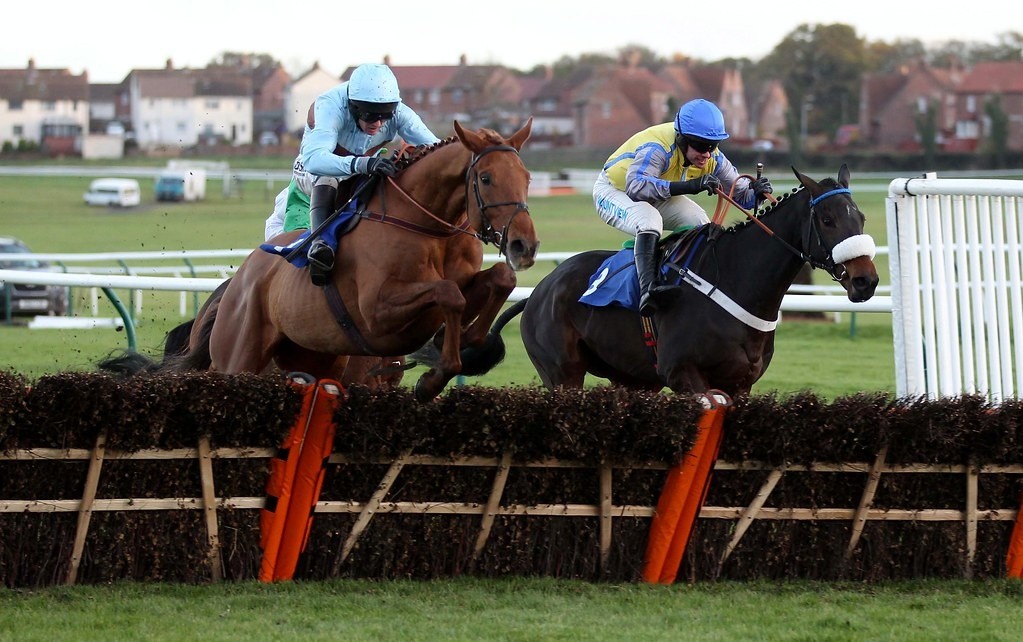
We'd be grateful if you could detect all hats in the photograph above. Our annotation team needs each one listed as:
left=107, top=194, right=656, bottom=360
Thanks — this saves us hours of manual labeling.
left=348, top=64, right=402, bottom=113
left=674, top=99, right=730, bottom=140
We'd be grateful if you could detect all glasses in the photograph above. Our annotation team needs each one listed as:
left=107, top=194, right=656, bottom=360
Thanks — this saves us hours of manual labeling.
left=347, top=85, right=396, bottom=123
left=678, top=113, right=723, bottom=154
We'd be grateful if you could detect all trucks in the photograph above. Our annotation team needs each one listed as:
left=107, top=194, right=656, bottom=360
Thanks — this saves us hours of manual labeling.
left=155, top=168, right=206, bottom=203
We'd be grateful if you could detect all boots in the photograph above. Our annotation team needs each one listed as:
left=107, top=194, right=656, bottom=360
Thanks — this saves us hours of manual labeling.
left=634, top=230, right=680, bottom=317
left=310, top=185, right=336, bottom=286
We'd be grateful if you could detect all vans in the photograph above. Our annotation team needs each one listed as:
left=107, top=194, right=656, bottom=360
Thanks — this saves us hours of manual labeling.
left=83, top=178, right=141, bottom=208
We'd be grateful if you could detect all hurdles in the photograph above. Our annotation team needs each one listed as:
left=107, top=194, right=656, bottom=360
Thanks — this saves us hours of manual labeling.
left=0, top=366, right=1023, bottom=589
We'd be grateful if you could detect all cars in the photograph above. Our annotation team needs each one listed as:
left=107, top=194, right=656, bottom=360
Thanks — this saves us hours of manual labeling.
left=0, top=235, right=68, bottom=322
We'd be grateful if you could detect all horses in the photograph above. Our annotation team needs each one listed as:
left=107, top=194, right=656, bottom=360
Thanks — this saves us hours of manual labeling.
left=93, top=114, right=541, bottom=406
left=484, top=164, right=881, bottom=400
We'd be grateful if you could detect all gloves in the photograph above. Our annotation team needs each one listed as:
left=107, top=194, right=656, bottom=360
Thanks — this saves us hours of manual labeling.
left=670, top=173, right=720, bottom=196
left=351, top=156, right=398, bottom=180
left=749, top=177, right=773, bottom=201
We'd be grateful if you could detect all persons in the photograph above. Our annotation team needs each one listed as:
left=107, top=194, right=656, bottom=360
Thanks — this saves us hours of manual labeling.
left=265, top=62, right=441, bottom=286
left=593, top=97, right=773, bottom=316
